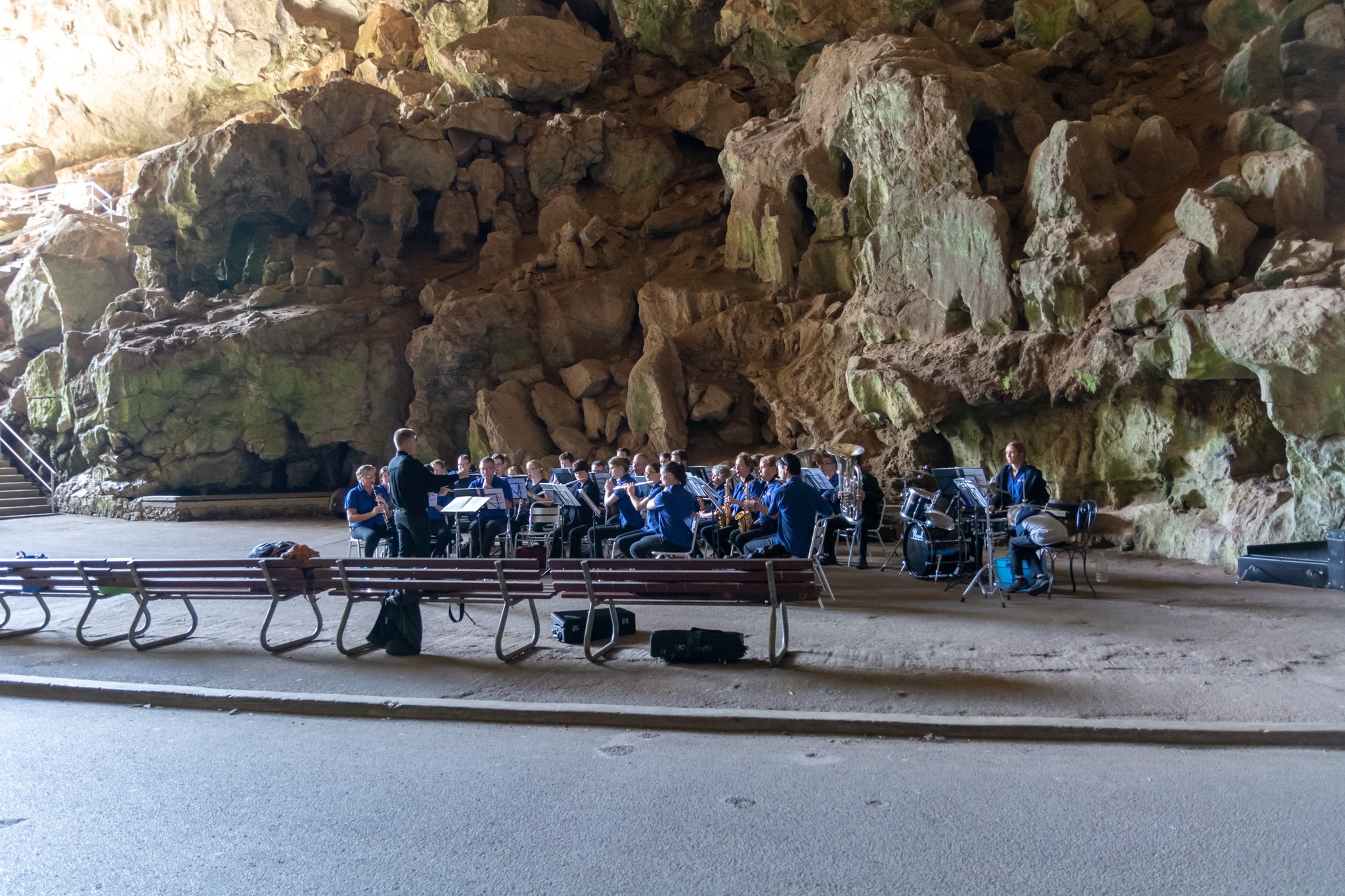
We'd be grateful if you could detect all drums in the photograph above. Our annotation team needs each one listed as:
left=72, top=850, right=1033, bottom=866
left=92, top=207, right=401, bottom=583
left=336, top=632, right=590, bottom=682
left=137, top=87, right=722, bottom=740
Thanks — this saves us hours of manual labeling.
left=898, top=486, right=937, bottom=525
left=902, top=520, right=976, bottom=581
left=971, top=517, right=1010, bottom=537
left=924, top=489, right=966, bottom=531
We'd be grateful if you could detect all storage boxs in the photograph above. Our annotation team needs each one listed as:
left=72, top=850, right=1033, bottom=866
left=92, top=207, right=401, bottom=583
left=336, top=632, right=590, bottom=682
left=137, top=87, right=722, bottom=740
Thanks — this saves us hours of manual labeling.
left=993, top=556, right=1047, bottom=592
left=550, top=607, right=636, bottom=644
left=649, top=627, right=749, bottom=665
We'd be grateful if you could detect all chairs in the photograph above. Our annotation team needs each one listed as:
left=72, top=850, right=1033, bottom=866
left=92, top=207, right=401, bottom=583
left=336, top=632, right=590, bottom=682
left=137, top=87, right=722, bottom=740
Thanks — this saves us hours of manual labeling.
left=807, top=516, right=836, bottom=609
left=429, top=497, right=741, bottom=568
left=347, top=518, right=394, bottom=559
left=1039, top=499, right=1098, bottom=601
left=834, top=497, right=891, bottom=568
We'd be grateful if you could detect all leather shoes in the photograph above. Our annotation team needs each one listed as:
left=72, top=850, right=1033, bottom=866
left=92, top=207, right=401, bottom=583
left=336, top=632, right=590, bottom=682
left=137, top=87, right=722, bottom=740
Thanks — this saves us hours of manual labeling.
left=856, top=560, right=868, bottom=568
left=1028, top=576, right=1051, bottom=595
left=1005, top=578, right=1029, bottom=592
left=819, top=556, right=838, bottom=564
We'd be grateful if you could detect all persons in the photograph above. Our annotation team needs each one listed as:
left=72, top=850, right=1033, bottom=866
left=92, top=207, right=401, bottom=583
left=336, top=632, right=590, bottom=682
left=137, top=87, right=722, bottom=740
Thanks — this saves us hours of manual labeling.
left=700, top=452, right=763, bottom=558
left=957, top=441, right=1051, bottom=569
left=615, top=462, right=662, bottom=558
left=729, top=454, right=782, bottom=557
left=378, top=448, right=838, bottom=545
left=820, top=456, right=884, bottom=569
left=545, top=459, right=600, bottom=572
left=343, top=464, right=398, bottom=558
left=467, top=455, right=513, bottom=558
left=1007, top=505, right=1068, bottom=596
left=587, top=456, right=643, bottom=558
left=387, top=428, right=467, bottom=559
left=423, top=465, right=452, bottom=558
left=743, top=453, right=834, bottom=560
left=690, top=465, right=731, bottom=558
left=630, top=460, right=693, bottom=559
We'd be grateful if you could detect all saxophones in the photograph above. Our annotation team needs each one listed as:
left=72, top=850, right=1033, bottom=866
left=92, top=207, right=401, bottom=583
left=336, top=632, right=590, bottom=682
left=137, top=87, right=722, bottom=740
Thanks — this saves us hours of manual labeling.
left=734, top=475, right=762, bottom=534
left=696, top=481, right=714, bottom=514
left=715, top=472, right=740, bottom=528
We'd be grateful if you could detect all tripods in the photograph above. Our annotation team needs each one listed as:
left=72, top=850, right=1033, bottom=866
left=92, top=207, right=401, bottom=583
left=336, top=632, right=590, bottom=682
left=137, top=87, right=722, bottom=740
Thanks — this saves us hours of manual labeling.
left=949, top=475, right=991, bottom=593
left=960, top=494, right=1011, bottom=608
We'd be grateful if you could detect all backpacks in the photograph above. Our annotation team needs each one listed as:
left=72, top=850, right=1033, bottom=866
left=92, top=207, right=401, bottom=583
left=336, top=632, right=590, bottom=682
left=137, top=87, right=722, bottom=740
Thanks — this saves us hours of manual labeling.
left=248, top=540, right=321, bottom=603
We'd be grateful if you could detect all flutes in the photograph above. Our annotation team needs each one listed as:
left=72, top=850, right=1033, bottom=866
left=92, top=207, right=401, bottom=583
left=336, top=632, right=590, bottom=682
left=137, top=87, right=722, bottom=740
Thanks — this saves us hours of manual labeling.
left=612, top=480, right=654, bottom=490
left=514, top=474, right=531, bottom=522
left=370, top=481, right=393, bottom=532
left=608, top=474, right=614, bottom=482
left=562, top=478, right=586, bottom=486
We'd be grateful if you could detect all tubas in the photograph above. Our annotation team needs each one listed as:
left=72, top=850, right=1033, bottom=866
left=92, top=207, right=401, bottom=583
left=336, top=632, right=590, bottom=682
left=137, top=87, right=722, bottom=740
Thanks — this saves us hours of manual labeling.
left=792, top=449, right=817, bottom=468
left=825, top=443, right=866, bottom=524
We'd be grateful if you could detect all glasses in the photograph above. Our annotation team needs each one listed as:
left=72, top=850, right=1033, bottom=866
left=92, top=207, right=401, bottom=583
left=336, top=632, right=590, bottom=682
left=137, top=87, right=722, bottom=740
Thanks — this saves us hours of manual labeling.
left=819, top=461, right=834, bottom=466
left=712, top=473, right=717, bottom=478
left=591, top=470, right=604, bottom=473
left=362, top=475, right=378, bottom=479
left=525, top=467, right=541, bottom=474
left=643, top=471, right=656, bottom=476
left=631, top=461, right=645, bottom=466
left=609, top=466, right=617, bottom=471
left=495, top=463, right=502, bottom=468
left=457, top=462, right=470, bottom=466
left=479, top=466, right=495, bottom=471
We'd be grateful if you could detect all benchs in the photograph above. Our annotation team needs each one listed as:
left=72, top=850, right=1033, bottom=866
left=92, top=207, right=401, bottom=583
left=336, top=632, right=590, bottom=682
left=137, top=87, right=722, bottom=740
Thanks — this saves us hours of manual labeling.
left=106, top=557, right=329, bottom=652
left=0, top=557, right=151, bottom=647
left=547, top=558, right=823, bottom=668
left=309, top=557, right=562, bottom=661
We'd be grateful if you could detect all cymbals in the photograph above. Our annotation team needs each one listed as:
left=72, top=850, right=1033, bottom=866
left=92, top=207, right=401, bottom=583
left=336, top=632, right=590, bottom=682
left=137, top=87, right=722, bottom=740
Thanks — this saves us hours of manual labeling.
left=901, top=466, right=935, bottom=478
left=965, top=481, right=1013, bottom=497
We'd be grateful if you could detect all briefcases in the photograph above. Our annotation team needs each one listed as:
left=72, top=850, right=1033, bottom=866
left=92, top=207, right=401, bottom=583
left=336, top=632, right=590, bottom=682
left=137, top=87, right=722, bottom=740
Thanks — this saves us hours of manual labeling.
left=650, top=627, right=747, bottom=665
left=550, top=606, right=635, bottom=643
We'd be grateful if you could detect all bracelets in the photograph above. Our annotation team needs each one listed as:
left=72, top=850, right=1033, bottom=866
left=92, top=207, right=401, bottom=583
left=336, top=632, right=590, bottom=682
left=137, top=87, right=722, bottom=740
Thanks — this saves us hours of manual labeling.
left=752, top=506, right=755, bottom=512
left=753, top=502, right=757, bottom=506
